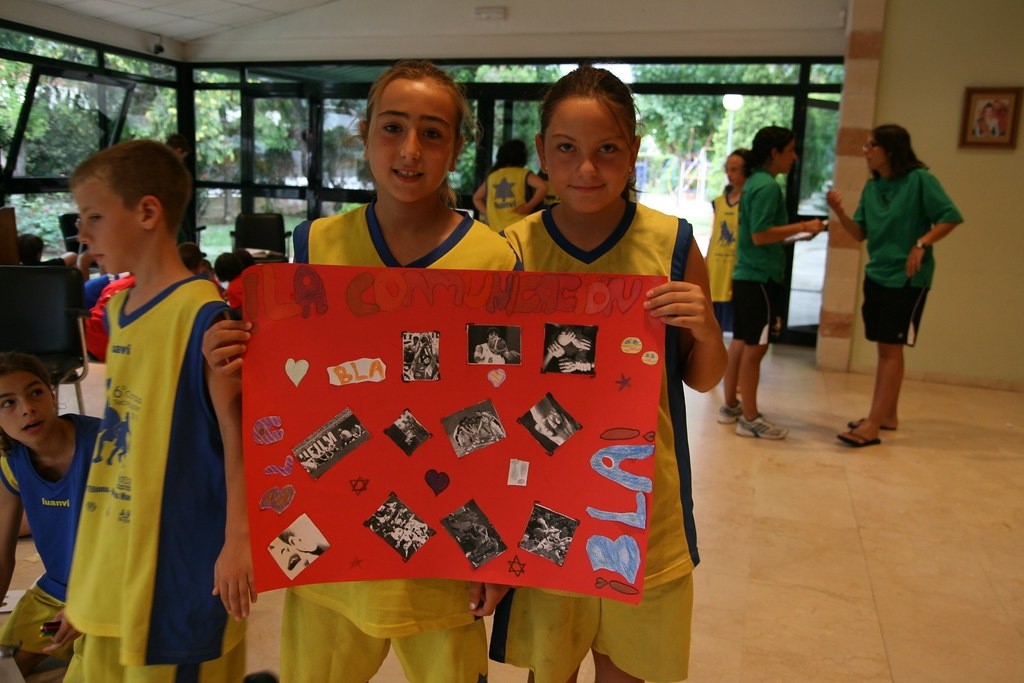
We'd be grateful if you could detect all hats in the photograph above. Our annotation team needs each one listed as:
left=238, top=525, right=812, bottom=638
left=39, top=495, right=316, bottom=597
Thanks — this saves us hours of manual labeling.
left=178, top=243, right=207, bottom=265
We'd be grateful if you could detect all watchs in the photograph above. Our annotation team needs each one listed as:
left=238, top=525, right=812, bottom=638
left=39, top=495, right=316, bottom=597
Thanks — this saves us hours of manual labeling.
left=916, top=240, right=926, bottom=248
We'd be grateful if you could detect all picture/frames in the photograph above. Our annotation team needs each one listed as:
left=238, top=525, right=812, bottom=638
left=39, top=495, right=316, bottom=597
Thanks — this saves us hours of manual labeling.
left=958, top=86, right=1024, bottom=150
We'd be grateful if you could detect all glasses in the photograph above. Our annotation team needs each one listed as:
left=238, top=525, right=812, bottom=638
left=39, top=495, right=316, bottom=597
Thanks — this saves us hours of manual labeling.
left=862, top=140, right=879, bottom=150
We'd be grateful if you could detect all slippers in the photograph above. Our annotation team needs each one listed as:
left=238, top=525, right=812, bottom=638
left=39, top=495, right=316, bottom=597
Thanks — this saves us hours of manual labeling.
left=848, top=418, right=897, bottom=431
left=837, top=428, right=880, bottom=448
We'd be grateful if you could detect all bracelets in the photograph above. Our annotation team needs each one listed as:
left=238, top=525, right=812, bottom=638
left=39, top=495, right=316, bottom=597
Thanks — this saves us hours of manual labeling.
left=802, top=222, right=806, bottom=231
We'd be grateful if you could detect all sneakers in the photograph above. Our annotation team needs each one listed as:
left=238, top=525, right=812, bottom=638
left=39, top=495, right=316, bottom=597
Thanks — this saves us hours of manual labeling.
left=734, top=414, right=789, bottom=439
left=717, top=399, right=742, bottom=424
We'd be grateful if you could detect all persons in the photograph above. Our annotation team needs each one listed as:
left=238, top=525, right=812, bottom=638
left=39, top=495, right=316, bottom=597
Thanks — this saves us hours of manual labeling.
left=472, top=137, right=561, bottom=233
left=300, top=423, right=366, bottom=472
left=530, top=396, right=576, bottom=445
left=202, top=63, right=524, bottom=683
left=706, top=148, right=750, bottom=394
left=827, top=123, right=964, bottom=447
left=404, top=332, right=439, bottom=381
left=0, top=352, right=103, bottom=676
left=544, top=328, right=594, bottom=373
left=402, top=415, right=429, bottom=441
left=973, top=101, right=1008, bottom=138
left=62, top=136, right=258, bottom=683
left=488, top=66, right=727, bottom=683
left=453, top=410, right=506, bottom=455
left=16, top=131, right=256, bottom=363
left=474, top=327, right=520, bottom=364
left=718, top=126, right=826, bottom=439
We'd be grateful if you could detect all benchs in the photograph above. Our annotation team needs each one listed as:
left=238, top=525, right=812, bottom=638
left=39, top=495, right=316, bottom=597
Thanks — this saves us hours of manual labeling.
left=0, top=264, right=93, bottom=417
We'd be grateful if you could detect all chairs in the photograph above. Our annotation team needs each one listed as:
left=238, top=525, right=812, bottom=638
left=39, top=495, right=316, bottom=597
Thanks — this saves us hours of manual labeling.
left=58, top=213, right=100, bottom=274
left=234, top=212, right=288, bottom=265
